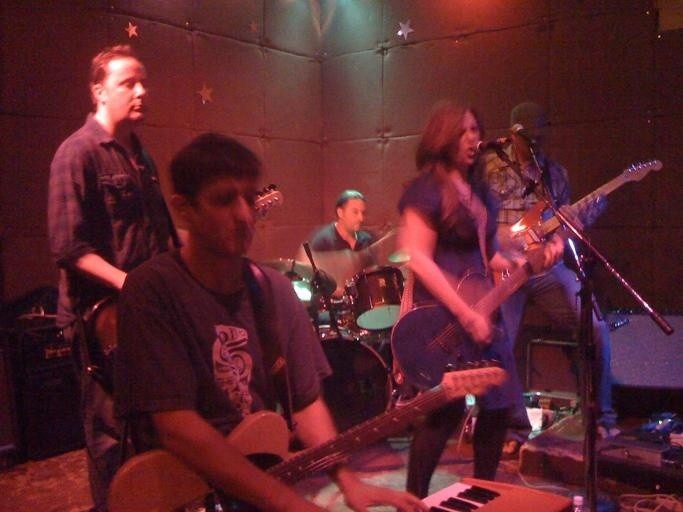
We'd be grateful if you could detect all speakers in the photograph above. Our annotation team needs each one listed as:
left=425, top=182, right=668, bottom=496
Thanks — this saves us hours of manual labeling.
left=606, top=311, right=683, bottom=413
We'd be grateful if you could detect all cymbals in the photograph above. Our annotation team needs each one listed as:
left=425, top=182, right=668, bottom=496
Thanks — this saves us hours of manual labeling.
left=261, top=258, right=339, bottom=297
left=386, top=249, right=411, bottom=263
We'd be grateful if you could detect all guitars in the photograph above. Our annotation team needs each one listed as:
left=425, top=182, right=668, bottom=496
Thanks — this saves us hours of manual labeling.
left=110, top=361, right=508, bottom=506
left=391, top=192, right=608, bottom=389
left=491, top=159, right=664, bottom=288
left=79, top=182, right=285, bottom=391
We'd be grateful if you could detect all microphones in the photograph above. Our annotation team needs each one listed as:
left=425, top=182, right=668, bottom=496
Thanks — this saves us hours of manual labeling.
left=476, top=137, right=509, bottom=153
left=512, top=124, right=535, bottom=145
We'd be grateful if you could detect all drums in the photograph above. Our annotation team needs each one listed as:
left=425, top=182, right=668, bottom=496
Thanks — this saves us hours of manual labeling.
left=314, top=327, right=394, bottom=430
left=350, top=267, right=403, bottom=328
left=332, top=293, right=395, bottom=356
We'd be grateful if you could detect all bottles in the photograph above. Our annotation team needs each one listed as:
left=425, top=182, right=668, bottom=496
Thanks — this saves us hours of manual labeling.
left=568, top=494, right=587, bottom=512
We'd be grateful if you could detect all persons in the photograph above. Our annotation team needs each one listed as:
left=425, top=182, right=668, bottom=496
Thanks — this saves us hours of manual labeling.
left=311, top=190, right=377, bottom=275
left=46, top=43, right=184, bottom=511
left=392, top=101, right=565, bottom=500
left=116, top=131, right=425, bottom=510
left=483, top=102, right=621, bottom=438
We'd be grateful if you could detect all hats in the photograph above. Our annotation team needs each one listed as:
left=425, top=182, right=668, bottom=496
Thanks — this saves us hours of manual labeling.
left=509, top=101, right=551, bottom=131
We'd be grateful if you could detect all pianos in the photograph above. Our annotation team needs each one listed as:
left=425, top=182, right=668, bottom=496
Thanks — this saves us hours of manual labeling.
left=398, top=477, right=574, bottom=507
